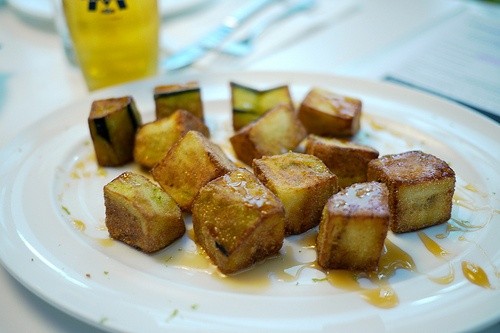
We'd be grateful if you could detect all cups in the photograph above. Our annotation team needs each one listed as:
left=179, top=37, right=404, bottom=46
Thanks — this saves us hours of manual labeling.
left=64, top=1, right=159, bottom=92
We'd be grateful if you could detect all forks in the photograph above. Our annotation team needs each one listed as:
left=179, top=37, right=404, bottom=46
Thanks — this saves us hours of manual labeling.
left=201, top=0, right=317, bottom=56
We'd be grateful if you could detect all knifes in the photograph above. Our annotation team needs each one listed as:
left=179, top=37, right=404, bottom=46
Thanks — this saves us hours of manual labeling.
left=160, top=0, right=273, bottom=72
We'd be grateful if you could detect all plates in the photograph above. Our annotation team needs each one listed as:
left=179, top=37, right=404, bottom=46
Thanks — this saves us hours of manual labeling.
left=0, top=66, right=500, bottom=333
